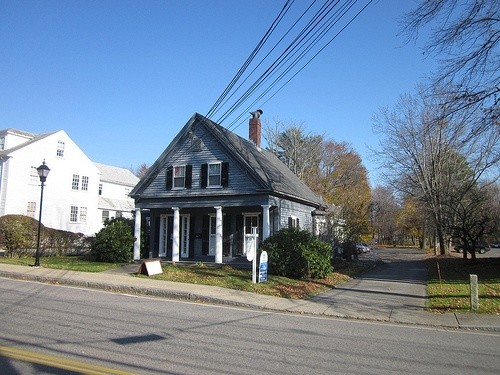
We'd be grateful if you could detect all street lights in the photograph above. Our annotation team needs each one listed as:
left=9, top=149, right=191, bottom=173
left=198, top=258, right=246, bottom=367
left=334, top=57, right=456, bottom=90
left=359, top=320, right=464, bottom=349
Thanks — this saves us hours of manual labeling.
left=34, top=161, right=50, bottom=266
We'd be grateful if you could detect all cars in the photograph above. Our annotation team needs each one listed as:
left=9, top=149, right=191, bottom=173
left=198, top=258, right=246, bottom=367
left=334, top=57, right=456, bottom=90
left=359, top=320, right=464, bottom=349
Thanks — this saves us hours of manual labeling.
left=354, top=243, right=371, bottom=254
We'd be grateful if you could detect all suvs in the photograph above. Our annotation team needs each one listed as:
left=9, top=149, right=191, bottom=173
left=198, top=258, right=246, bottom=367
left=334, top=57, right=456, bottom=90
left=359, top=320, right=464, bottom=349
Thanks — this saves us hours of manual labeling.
left=454, top=242, right=491, bottom=253
